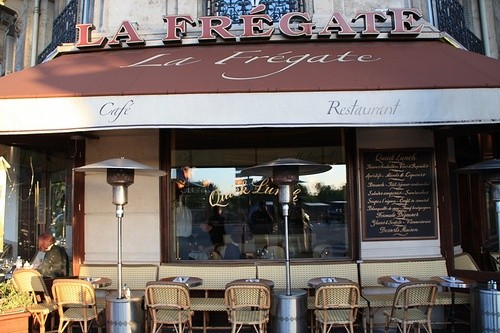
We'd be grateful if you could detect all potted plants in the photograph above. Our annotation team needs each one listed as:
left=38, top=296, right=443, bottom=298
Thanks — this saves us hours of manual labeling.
left=0, top=279, right=31, bottom=333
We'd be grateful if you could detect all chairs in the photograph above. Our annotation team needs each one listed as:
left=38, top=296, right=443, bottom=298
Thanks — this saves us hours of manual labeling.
left=13, top=269, right=65, bottom=333
left=145, top=281, right=194, bottom=333
left=384, top=280, right=441, bottom=333
left=313, top=281, right=360, bottom=333
left=207, top=243, right=333, bottom=258
left=225, top=283, right=271, bottom=333
left=51, top=278, right=105, bottom=333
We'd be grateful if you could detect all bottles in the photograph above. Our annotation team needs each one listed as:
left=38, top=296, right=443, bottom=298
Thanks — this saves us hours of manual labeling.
left=256, top=248, right=275, bottom=259
left=122, top=283, right=131, bottom=299
left=488, top=280, right=497, bottom=291
left=24, top=261, right=31, bottom=269
left=16, top=256, right=22, bottom=269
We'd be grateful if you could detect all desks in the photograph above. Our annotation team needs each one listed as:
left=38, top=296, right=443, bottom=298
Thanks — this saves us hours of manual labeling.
left=225, top=278, right=274, bottom=333
left=51, top=276, right=112, bottom=333
left=377, top=276, right=427, bottom=333
left=307, top=277, right=358, bottom=333
left=430, top=275, right=477, bottom=333
left=159, top=277, right=203, bottom=333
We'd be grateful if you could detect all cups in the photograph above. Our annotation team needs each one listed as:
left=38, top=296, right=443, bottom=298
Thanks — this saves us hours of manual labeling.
left=2, top=258, right=12, bottom=273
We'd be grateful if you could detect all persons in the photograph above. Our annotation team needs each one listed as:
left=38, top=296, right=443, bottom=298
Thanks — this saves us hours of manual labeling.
left=207, top=205, right=226, bottom=251
left=249, top=200, right=274, bottom=237
left=31, top=232, right=69, bottom=276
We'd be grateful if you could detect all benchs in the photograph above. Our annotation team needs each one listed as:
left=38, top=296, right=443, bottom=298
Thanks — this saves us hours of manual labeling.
left=78, top=258, right=473, bottom=333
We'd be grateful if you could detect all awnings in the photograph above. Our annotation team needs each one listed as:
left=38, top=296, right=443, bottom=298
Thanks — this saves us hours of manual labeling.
left=0, top=39, right=500, bottom=156
